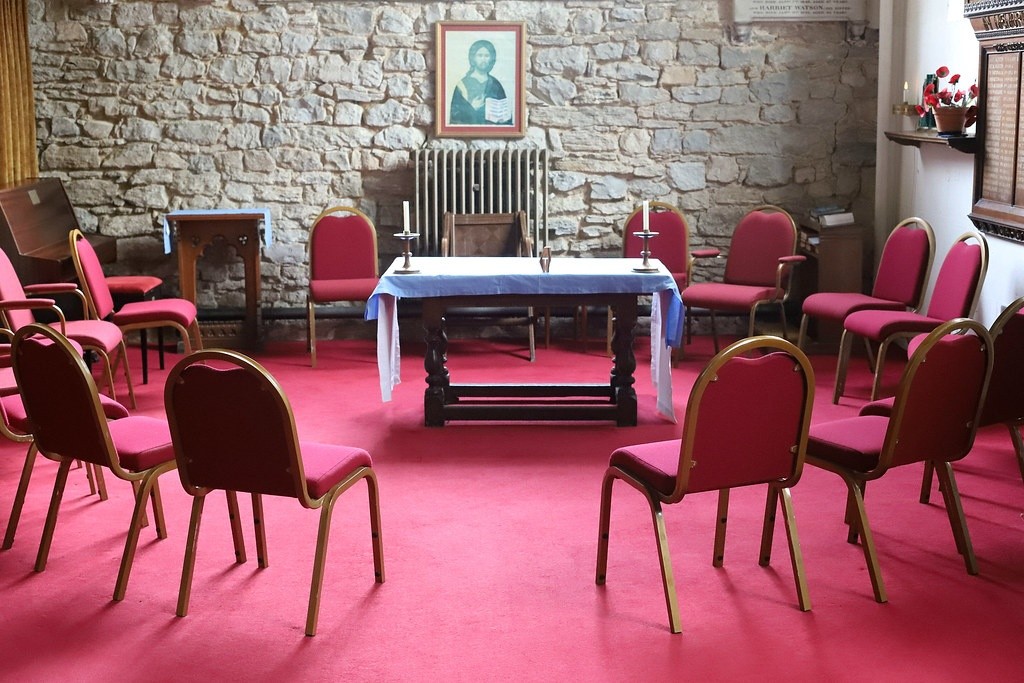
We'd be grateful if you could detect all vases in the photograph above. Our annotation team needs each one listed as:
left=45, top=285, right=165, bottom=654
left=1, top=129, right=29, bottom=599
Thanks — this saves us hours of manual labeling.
left=934, top=108, right=966, bottom=136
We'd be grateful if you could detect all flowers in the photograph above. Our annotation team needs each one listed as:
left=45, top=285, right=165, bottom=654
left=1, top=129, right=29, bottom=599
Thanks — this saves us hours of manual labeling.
left=916, top=68, right=978, bottom=115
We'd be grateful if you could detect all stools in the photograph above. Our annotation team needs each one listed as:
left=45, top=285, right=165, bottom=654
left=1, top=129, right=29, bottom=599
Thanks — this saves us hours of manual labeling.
left=102, top=274, right=164, bottom=383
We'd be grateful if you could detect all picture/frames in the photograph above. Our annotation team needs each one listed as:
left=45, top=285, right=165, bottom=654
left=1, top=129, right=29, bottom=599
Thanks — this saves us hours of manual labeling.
left=434, top=20, right=526, bottom=138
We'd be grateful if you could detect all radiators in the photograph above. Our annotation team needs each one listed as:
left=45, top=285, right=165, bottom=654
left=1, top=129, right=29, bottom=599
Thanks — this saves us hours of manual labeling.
left=411, top=148, right=549, bottom=256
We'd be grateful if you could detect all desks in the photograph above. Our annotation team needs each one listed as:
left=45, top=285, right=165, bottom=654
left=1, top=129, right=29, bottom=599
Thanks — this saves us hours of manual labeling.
left=363, top=256, right=684, bottom=427
left=164, top=212, right=266, bottom=351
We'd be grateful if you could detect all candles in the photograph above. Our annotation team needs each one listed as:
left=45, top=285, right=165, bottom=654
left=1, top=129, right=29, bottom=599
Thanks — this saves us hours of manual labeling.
left=402, top=200, right=409, bottom=231
left=642, top=201, right=649, bottom=230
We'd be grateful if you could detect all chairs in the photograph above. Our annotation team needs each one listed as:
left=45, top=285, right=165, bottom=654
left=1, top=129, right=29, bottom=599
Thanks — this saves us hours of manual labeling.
left=593, top=203, right=1024, bottom=635
left=0, top=209, right=394, bottom=635
